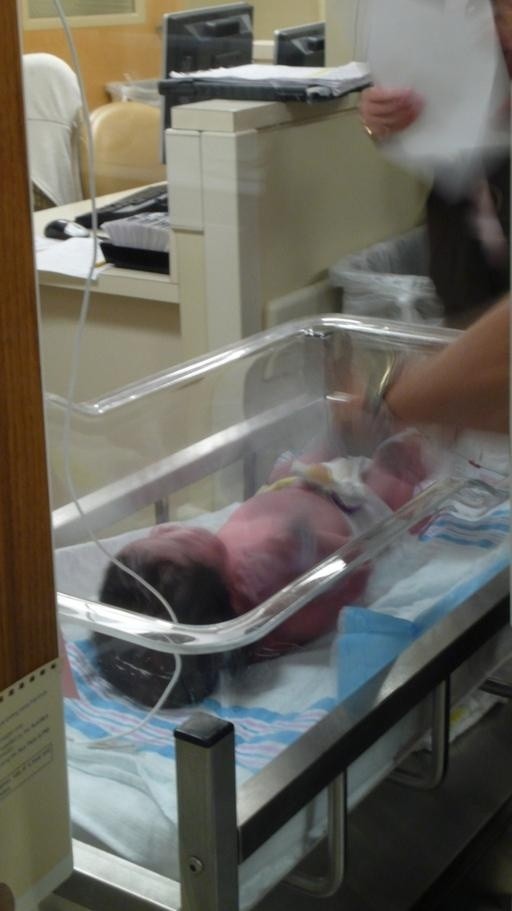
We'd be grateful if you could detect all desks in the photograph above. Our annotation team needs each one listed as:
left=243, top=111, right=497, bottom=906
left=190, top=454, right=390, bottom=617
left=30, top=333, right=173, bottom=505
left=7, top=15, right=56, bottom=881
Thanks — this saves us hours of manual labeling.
left=32, top=181, right=180, bottom=509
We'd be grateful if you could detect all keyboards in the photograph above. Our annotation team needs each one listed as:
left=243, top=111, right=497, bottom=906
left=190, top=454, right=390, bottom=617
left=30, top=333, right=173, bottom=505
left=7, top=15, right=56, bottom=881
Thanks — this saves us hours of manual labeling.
left=73, top=181, right=167, bottom=226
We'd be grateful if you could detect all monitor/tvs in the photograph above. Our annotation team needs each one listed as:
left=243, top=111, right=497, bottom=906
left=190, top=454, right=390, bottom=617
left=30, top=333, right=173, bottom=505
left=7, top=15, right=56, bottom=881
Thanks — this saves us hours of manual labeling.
left=275, top=19, right=327, bottom=67
left=159, top=1, right=254, bottom=165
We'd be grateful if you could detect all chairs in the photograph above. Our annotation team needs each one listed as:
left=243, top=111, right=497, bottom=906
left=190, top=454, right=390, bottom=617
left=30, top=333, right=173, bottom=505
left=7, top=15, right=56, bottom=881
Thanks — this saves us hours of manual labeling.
left=77, top=101, right=170, bottom=202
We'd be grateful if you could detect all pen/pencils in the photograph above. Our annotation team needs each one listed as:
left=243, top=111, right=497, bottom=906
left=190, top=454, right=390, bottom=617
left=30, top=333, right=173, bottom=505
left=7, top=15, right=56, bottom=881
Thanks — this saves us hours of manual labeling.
left=94, top=262, right=107, bottom=268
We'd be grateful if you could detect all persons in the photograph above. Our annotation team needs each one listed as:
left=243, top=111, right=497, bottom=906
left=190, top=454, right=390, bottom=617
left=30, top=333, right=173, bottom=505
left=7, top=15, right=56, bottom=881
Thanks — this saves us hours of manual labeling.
left=356, top=1, right=512, bottom=327
left=93, top=418, right=458, bottom=708
left=364, top=292, right=511, bottom=432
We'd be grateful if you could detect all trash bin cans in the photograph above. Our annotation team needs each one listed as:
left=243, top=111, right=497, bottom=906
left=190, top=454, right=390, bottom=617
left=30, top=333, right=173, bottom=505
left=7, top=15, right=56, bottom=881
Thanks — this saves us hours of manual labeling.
left=328, top=223, right=444, bottom=458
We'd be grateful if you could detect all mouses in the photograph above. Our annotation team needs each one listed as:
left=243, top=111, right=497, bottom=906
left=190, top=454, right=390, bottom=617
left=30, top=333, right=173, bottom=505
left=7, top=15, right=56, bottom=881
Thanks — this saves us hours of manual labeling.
left=43, top=217, right=89, bottom=242
